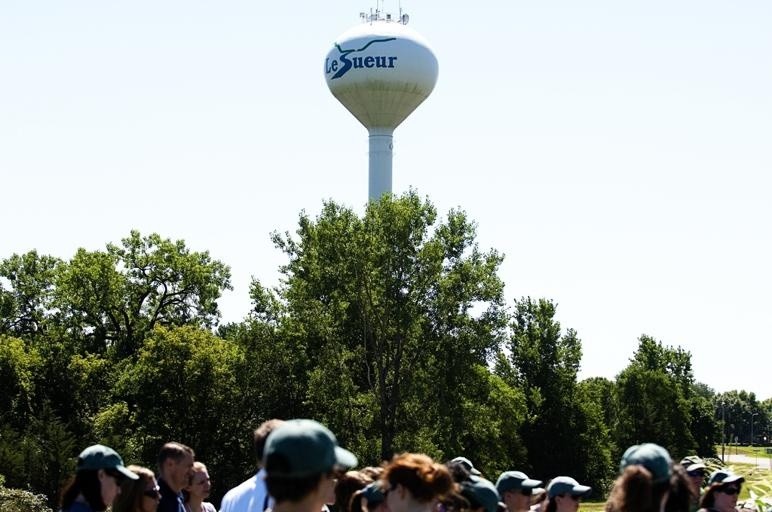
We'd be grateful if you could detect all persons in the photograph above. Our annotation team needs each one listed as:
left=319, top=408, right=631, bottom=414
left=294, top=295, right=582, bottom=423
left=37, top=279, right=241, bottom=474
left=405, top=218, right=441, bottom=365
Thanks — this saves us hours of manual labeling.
left=221, top=418, right=592, bottom=512
left=156, top=442, right=194, bottom=512
left=58, top=445, right=137, bottom=511
left=182, top=459, right=216, bottom=511
left=112, top=464, right=162, bottom=511
left=605, top=442, right=747, bottom=512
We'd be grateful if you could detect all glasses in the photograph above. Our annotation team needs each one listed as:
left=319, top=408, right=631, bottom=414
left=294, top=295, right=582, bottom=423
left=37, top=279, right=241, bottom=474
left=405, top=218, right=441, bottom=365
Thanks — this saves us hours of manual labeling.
left=141, top=487, right=160, bottom=499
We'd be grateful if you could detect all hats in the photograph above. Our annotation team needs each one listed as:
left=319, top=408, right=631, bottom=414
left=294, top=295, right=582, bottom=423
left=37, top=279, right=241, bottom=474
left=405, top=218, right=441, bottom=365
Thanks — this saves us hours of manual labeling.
left=77, top=444, right=140, bottom=480
left=621, top=442, right=746, bottom=495
left=262, top=418, right=359, bottom=469
left=447, top=456, right=593, bottom=511
